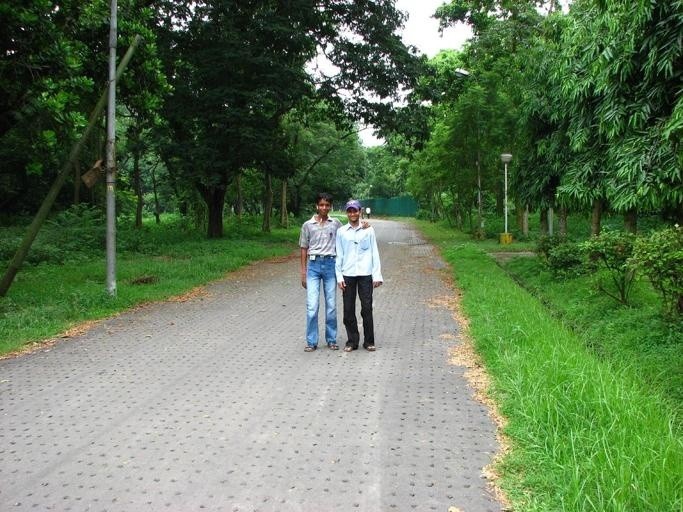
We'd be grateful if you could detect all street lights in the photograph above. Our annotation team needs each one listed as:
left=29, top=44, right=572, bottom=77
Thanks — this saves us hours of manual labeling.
left=497, top=153, right=513, bottom=244
left=452, top=66, right=485, bottom=240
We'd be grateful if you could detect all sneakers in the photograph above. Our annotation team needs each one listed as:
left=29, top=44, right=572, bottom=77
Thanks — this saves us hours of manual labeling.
left=328, top=345, right=339, bottom=350
left=344, top=345, right=353, bottom=352
left=304, top=346, right=317, bottom=351
left=367, top=345, right=375, bottom=351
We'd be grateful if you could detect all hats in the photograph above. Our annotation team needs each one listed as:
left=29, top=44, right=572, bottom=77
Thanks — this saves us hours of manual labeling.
left=345, top=200, right=361, bottom=210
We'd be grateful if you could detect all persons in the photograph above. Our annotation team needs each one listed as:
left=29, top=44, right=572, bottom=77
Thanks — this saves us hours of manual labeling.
left=361, top=206, right=365, bottom=219
left=298, top=193, right=371, bottom=352
left=334, top=199, right=384, bottom=352
left=366, top=206, right=371, bottom=219
